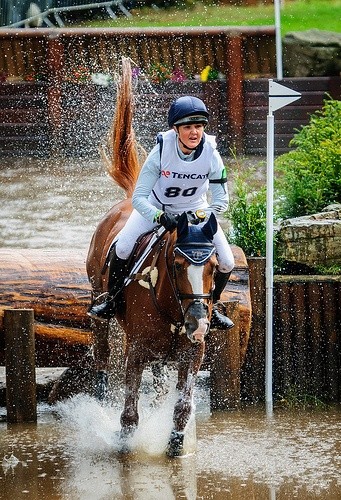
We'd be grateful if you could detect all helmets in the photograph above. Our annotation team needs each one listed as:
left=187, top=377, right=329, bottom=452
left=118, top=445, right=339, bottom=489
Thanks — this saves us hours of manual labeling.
left=168, top=96, right=210, bottom=127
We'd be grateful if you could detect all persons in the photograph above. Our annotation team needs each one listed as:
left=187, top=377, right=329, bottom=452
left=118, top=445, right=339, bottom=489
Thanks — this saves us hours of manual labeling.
left=87, top=96, right=234, bottom=330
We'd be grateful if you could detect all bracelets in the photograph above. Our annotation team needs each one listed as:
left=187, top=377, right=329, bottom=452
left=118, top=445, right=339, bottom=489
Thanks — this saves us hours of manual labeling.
left=157, top=212, right=164, bottom=224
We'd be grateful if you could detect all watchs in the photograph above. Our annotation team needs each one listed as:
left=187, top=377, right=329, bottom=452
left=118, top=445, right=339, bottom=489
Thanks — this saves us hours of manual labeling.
left=195, top=210, right=206, bottom=223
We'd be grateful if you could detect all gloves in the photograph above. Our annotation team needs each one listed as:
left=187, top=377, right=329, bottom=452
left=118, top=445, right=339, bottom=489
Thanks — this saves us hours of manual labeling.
left=160, top=212, right=176, bottom=231
left=179, top=211, right=195, bottom=228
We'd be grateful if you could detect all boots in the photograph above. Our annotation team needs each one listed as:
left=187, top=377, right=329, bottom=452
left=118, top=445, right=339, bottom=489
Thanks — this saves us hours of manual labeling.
left=210, top=267, right=235, bottom=329
left=86, top=253, right=128, bottom=321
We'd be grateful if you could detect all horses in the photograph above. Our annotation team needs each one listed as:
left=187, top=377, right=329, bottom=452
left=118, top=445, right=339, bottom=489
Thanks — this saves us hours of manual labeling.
left=48, top=55, right=219, bottom=459
